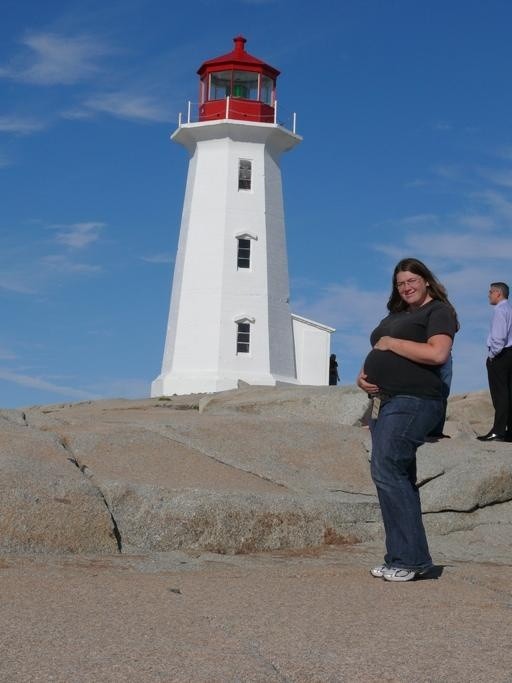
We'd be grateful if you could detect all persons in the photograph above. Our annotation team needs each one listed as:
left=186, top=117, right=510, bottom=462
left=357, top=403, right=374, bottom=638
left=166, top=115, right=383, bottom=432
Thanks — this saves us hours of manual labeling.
left=425, top=284, right=452, bottom=443
left=329, top=354, right=340, bottom=385
left=476, top=283, right=512, bottom=443
left=357, top=258, right=460, bottom=581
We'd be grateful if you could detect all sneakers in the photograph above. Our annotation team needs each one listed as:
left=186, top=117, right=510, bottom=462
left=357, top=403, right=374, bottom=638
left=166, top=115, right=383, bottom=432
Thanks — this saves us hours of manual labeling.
left=369, top=565, right=389, bottom=578
left=382, top=564, right=434, bottom=582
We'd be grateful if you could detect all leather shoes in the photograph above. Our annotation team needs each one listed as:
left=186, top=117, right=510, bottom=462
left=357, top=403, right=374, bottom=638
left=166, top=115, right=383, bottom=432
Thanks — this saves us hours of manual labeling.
left=477, top=430, right=504, bottom=441
